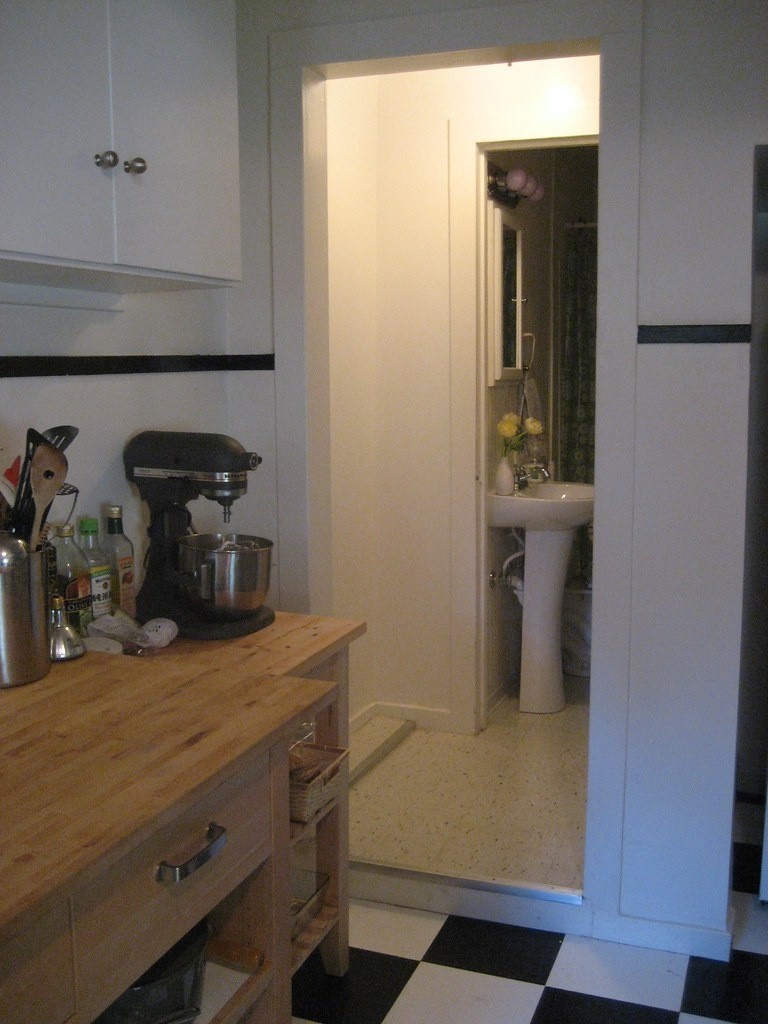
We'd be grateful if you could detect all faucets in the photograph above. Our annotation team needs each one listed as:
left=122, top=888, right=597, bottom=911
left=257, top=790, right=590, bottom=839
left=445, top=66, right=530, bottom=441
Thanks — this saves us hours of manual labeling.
left=514, top=463, right=551, bottom=484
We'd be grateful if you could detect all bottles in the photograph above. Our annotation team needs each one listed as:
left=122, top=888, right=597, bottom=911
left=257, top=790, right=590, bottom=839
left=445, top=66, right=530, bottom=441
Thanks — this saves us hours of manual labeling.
left=55, top=528, right=91, bottom=632
left=47, top=592, right=86, bottom=661
left=79, top=519, right=110, bottom=618
left=104, top=505, right=134, bottom=611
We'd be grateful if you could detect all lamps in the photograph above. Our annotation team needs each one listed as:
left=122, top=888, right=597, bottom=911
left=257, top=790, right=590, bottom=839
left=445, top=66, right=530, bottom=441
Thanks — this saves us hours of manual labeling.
left=487, top=161, right=544, bottom=209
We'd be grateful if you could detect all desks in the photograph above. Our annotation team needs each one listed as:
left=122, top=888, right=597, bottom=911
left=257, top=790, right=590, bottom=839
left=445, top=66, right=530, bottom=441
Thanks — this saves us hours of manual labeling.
left=0, top=609, right=369, bottom=1024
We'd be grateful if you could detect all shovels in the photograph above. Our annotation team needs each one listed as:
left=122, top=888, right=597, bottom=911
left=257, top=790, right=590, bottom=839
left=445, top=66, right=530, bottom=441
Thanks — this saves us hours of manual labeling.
left=11, top=425, right=80, bottom=552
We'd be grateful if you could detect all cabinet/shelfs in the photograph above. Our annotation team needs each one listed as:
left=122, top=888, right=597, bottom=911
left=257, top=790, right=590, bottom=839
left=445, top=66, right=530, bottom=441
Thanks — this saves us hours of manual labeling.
left=0, top=0, right=243, bottom=281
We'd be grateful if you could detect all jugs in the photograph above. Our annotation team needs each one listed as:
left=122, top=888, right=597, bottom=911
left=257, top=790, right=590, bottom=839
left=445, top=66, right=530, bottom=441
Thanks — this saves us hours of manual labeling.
left=524, top=460, right=546, bottom=483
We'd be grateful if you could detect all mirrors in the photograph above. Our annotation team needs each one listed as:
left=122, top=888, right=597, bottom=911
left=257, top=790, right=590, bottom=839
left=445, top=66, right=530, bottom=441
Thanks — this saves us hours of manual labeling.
left=487, top=200, right=524, bottom=388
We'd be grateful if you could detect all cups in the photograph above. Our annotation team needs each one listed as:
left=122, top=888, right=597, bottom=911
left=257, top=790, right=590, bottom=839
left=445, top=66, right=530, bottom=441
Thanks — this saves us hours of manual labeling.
left=0, top=547, right=52, bottom=688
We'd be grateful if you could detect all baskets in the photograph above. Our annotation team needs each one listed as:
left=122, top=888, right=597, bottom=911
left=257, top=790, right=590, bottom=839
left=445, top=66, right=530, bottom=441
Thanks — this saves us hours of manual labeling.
left=288, top=740, right=349, bottom=824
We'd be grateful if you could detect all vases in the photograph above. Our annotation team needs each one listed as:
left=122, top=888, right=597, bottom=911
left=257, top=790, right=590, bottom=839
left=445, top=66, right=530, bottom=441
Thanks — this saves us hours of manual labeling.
left=495, top=457, right=514, bottom=496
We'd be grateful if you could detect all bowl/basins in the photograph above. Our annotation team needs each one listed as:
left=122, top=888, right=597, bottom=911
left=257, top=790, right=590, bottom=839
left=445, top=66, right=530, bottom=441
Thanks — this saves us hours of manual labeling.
left=176, top=532, right=275, bottom=616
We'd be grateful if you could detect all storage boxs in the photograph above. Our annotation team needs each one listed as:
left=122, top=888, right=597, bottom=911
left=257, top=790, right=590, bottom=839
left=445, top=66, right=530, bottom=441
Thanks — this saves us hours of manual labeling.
left=289, top=744, right=349, bottom=823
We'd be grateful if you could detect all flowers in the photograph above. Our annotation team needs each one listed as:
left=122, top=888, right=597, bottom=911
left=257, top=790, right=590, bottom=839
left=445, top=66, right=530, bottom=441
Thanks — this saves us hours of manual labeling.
left=496, top=412, right=543, bottom=457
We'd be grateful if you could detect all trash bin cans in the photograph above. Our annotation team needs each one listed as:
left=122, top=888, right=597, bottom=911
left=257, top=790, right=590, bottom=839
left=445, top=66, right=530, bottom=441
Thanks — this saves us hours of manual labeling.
left=96, top=922, right=215, bottom=1024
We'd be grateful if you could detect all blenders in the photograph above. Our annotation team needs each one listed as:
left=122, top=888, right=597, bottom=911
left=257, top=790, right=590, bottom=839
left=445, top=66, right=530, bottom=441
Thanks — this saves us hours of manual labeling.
left=122, top=429, right=275, bottom=640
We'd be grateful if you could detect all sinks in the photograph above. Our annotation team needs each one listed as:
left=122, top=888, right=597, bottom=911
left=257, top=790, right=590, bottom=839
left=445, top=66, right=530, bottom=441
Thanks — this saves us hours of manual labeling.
left=488, top=481, right=594, bottom=527
